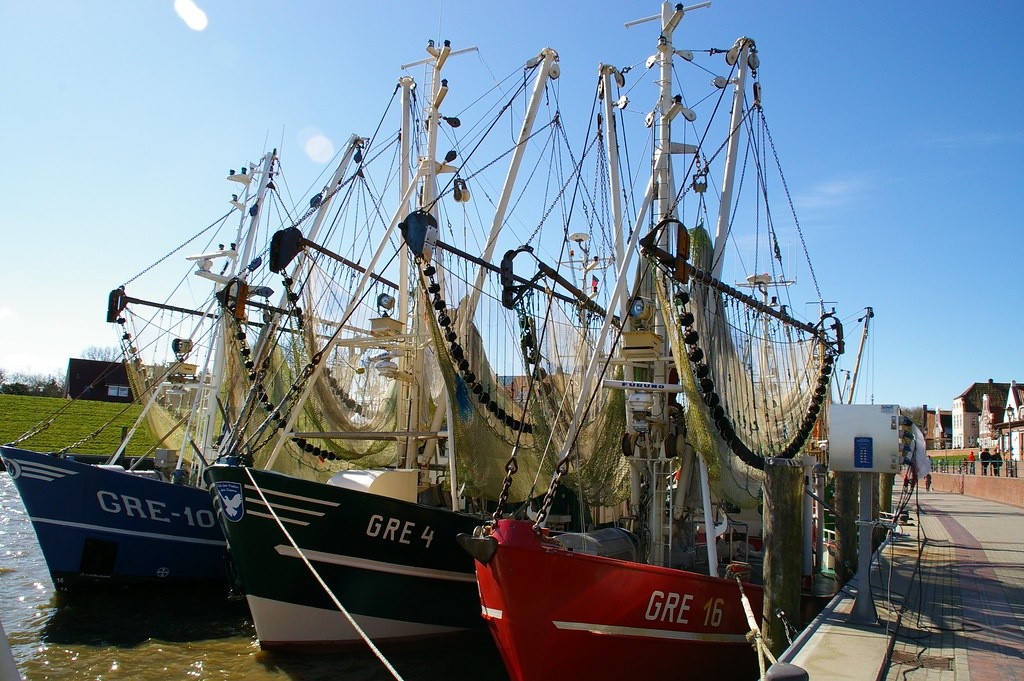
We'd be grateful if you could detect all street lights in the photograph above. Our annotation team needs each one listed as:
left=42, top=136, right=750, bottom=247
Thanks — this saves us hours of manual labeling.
left=1005, top=402, right=1017, bottom=476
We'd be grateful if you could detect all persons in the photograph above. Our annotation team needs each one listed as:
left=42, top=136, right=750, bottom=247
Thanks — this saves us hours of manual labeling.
left=924, top=474, right=931, bottom=494
left=938, top=447, right=1002, bottom=476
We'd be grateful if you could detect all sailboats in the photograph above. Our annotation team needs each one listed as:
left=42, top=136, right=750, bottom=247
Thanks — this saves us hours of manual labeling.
left=455, top=0, right=877, bottom=680
left=186, top=34, right=572, bottom=650
left=0, top=148, right=299, bottom=615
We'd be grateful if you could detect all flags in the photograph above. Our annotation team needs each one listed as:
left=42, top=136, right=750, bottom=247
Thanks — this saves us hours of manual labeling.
left=592, top=275, right=599, bottom=287
